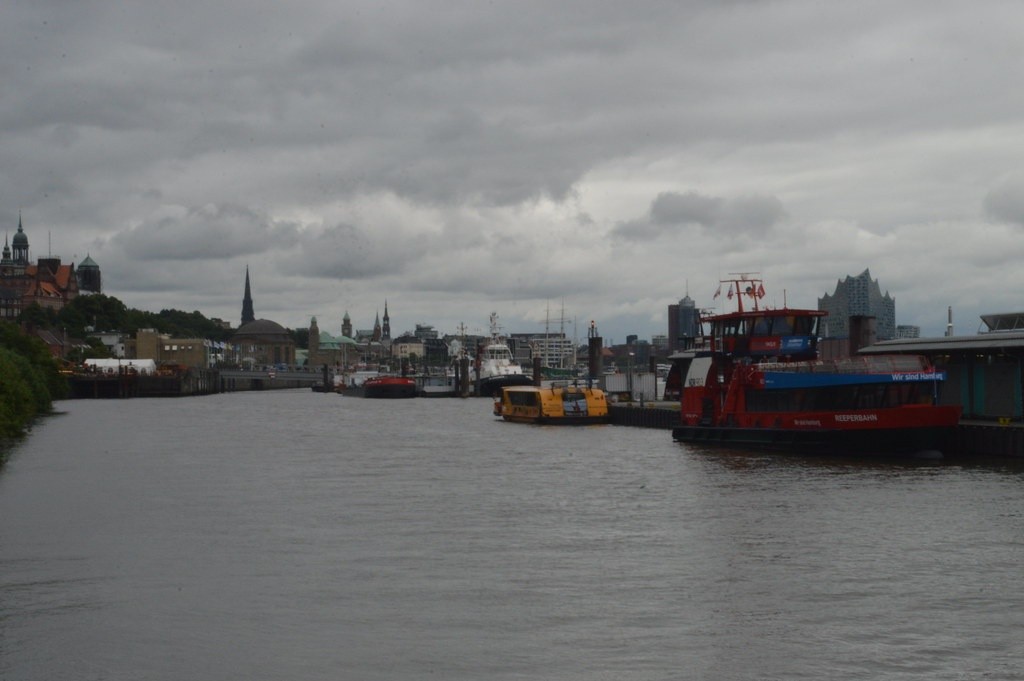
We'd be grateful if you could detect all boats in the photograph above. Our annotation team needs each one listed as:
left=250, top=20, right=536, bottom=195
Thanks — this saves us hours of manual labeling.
left=469, top=312, right=535, bottom=397
left=362, top=376, right=416, bottom=399
left=671, top=272, right=964, bottom=458
left=493, top=380, right=608, bottom=425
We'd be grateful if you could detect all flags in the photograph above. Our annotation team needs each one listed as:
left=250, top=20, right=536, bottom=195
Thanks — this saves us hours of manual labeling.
left=204, top=339, right=257, bottom=353
left=713, top=283, right=765, bottom=300
